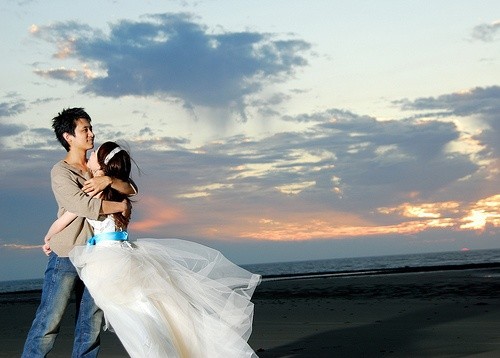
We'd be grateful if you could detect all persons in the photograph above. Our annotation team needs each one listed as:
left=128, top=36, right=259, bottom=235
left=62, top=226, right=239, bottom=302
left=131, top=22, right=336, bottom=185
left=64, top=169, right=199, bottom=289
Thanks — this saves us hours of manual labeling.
left=21, top=106, right=138, bottom=358
left=41, top=141, right=262, bottom=358
